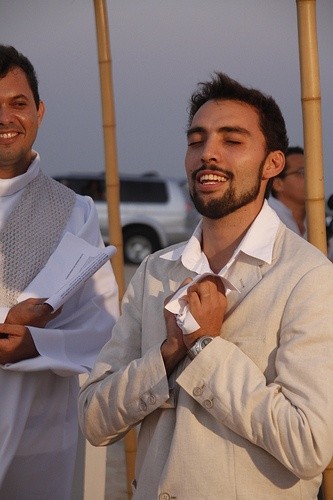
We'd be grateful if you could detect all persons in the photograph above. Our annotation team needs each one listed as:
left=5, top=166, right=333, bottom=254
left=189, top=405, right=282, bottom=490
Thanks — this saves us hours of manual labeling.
left=77, top=71, right=333, bottom=500
left=0, top=45, right=120, bottom=500
left=268, top=146, right=307, bottom=241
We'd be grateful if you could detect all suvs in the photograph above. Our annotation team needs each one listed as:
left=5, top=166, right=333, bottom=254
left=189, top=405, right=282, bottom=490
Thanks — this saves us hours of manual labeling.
left=49, top=170, right=189, bottom=263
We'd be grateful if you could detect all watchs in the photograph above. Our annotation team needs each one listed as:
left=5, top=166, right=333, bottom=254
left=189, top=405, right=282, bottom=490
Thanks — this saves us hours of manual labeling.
left=187, top=335, right=216, bottom=361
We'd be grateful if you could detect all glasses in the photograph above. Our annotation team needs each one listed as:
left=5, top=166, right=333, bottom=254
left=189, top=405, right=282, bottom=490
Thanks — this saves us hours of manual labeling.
left=285, top=166, right=305, bottom=177
